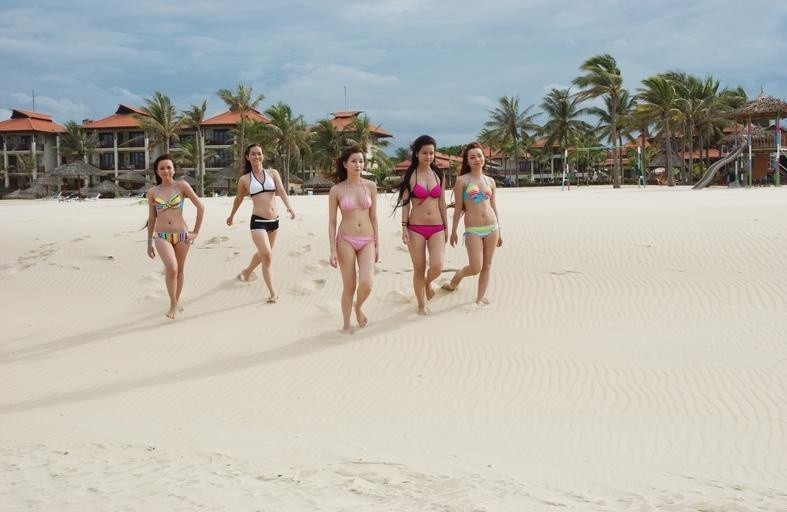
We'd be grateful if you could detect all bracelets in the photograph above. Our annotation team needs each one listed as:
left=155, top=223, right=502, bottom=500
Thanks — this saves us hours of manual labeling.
left=402, top=224, right=407, bottom=227
left=401, top=222, right=407, bottom=224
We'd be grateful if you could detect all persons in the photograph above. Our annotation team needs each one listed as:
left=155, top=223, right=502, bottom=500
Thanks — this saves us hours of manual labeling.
left=327, top=144, right=378, bottom=336
left=447, top=141, right=503, bottom=304
left=397, top=134, right=448, bottom=317
left=224, top=144, right=297, bottom=303
left=145, top=153, right=206, bottom=321
